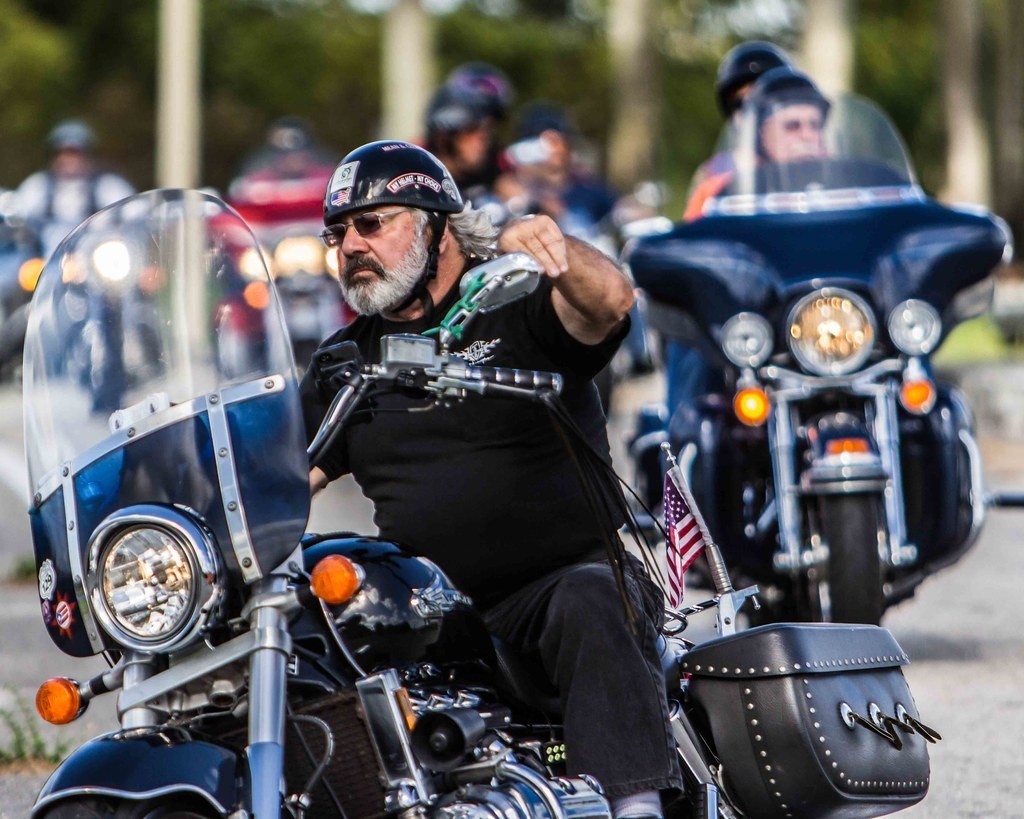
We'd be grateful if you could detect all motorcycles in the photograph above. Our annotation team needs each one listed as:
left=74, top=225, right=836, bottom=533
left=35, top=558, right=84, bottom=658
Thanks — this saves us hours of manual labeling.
left=20, top=184, right=944, bottom=819
left=0, top=88, right=1023, bottom=626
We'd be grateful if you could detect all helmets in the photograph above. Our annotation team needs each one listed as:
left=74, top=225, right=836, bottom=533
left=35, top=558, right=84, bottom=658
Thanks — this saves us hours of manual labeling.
left=716, top=41, right=790, bottom=120
left=750, top=65, right=830, bottom=114
left=47, top=120, right=97, bottom=153
left=266, top=126, right=308, bottom=152
left=322, top=139, right=463, bottom=226
left=428, top=85, right=509, bottom=132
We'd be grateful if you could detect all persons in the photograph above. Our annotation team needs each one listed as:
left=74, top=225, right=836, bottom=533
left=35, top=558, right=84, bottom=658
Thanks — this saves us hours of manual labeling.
left=242, top=138, right=691, bottom=819
left=0, top=43, right=833, bottom=470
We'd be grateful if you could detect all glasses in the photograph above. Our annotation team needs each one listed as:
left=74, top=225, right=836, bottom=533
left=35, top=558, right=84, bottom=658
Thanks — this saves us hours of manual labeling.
left=318, top=206, right=418, bottom=247
left=784, top=118, right=824, bottom=130
